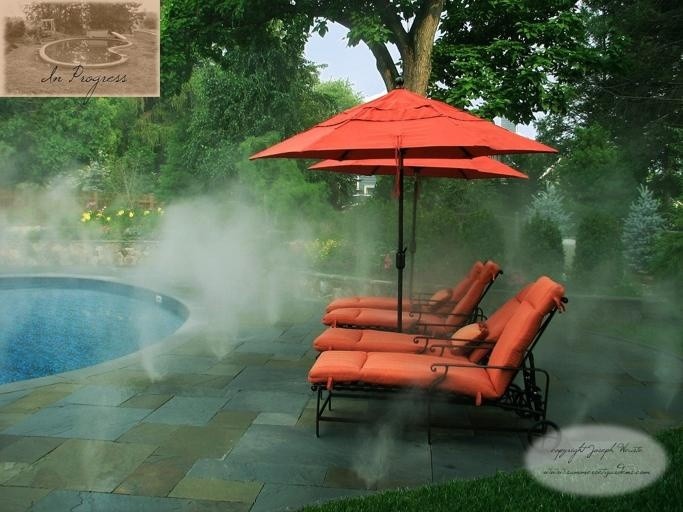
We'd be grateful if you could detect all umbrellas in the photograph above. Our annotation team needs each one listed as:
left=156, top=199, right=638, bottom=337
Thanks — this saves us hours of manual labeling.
left=249, top=76, right=558, bottom=337
left=309, top=157, right=529, bottom=305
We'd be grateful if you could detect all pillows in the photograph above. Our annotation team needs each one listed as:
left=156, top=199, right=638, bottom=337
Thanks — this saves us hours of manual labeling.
left=445, top=320, right=489, bottom=357
left=425, top=286, right=451, bottom=310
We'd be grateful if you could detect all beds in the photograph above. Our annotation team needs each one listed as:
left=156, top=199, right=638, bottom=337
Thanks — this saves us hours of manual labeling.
left=322, top=257, right=484, bottom=315
left=311, top=282, right=550, bottom=400
left=305, top=276, right=562, bottom=456
left=322, top=262, right=501, bottom=338
